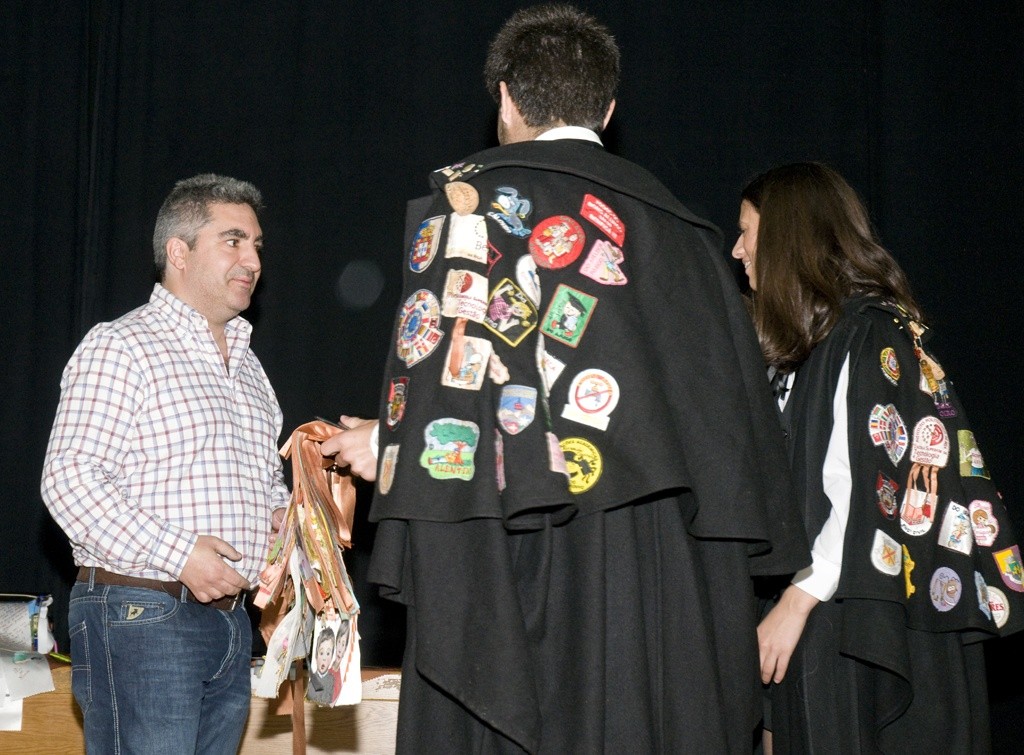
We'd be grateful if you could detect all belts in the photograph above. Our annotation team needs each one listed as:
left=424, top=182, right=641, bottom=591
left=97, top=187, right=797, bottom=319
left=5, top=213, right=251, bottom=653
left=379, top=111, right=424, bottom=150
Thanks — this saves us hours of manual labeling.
left=76, top=566, right=244, bottom=611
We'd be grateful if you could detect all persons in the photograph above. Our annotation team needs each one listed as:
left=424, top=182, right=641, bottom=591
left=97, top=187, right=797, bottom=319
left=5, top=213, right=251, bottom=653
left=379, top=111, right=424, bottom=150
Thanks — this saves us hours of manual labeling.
left=307, top=620, right=350, bottom=703
left=322, top=3, right=812, bottom=755
left=732, top=160, right=1024, bottom=755
left=41, top=173, right=297, bottom=755
